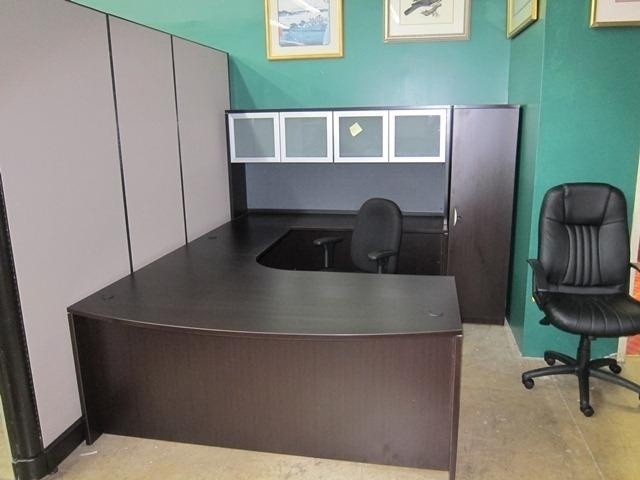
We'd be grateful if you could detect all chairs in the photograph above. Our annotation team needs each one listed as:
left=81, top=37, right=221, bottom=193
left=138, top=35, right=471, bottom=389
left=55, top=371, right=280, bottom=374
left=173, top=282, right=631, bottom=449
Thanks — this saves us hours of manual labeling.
left=309, top=196, right=404, bottom=276
left=519, top=180, right=639, bottom=418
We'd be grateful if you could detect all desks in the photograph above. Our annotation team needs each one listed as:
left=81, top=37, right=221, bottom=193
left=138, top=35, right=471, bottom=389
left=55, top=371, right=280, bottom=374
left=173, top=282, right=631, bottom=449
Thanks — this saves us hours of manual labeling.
left=62, top=207, right=465, bottom=479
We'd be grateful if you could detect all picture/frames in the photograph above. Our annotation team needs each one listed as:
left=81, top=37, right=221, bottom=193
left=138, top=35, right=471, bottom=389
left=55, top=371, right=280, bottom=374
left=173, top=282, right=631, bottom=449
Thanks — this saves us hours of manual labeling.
left=505, top=0, right=537, bottom=40
left=263, top=0, right=346, bottom=61
left=380, top=0, right=473, bottom=44
left=588, top=0, right=640, bottom=30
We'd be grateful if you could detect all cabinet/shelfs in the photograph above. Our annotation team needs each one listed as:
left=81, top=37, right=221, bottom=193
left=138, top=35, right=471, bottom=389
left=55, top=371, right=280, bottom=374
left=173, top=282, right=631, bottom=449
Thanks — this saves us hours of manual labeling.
left=332, top=105, right=451, bottom=165
left=443, top=102, right=525, bottom=327
left=225, top=107, right=335, bottom=165
